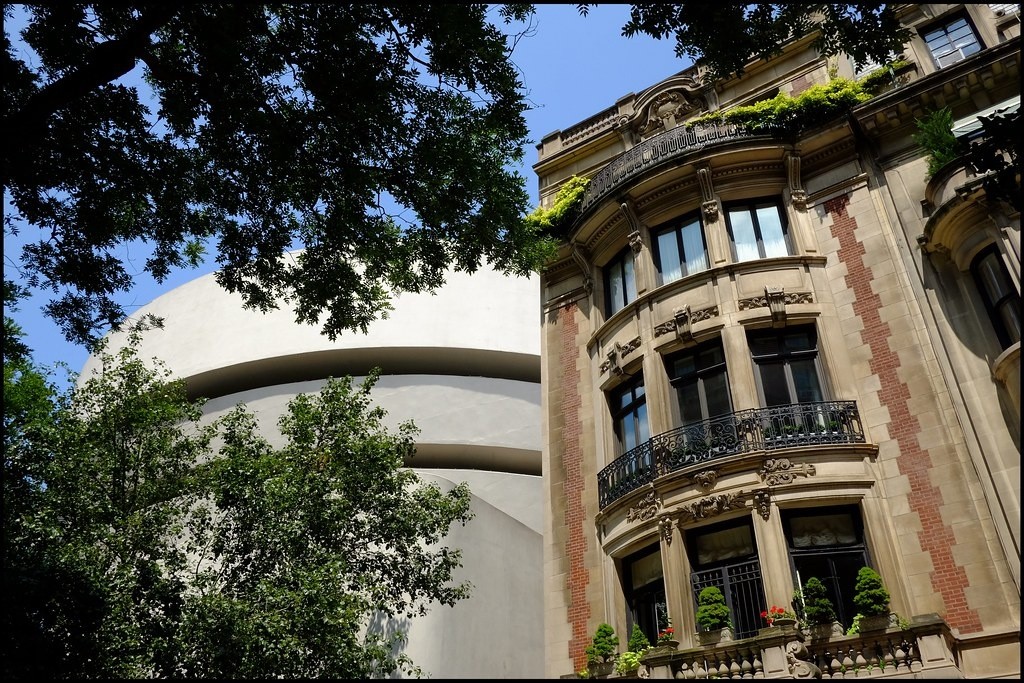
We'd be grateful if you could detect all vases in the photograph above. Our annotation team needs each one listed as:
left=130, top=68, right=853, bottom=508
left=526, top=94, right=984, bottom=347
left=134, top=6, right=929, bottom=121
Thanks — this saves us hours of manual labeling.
left=656, top=640, right=679, bottom=648
left=772, top=618, right=796, bottom=626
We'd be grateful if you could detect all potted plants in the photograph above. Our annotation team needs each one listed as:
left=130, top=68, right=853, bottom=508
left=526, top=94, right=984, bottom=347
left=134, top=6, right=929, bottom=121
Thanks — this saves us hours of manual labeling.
left=585, top=622, right=620, bottom=676
left=694, top=585, right=736, bottom=645
left=616, top=647, right=654, bottom=677
left=802, top=577, right=844, bottom=642
left=853, top=566, right=890, bottom=633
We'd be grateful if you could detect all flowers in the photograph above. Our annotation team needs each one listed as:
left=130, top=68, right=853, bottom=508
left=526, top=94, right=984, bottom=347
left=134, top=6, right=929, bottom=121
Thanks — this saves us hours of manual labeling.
left=655, top=601, right=674, bottom=642
left=761, top=605, right=797, bottom=624
